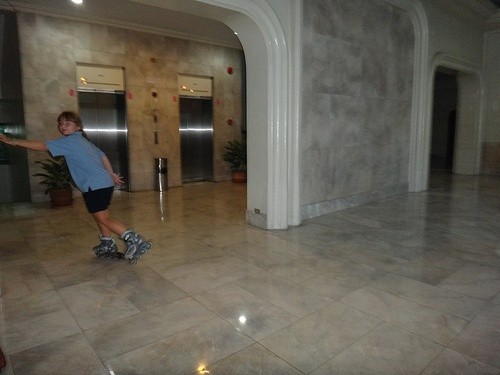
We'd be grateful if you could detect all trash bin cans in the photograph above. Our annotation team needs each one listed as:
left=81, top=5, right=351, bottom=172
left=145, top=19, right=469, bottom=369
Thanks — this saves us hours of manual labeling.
left=154, top=158, right=168, bottom=192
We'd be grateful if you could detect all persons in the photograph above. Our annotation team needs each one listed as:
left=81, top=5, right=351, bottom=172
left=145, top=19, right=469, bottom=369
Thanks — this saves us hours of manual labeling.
left=0, top=111, right=153, bottom=265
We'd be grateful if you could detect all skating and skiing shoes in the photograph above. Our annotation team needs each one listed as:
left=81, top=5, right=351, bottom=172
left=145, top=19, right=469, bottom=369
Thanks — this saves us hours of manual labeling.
left=93, top=238, right=122, bottom=260
left=119, top=230, right=153, bottom=264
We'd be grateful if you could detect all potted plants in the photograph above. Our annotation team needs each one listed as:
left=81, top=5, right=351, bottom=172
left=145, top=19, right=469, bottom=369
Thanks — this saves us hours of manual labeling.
left=223, top=139, right=247, bottom=183
left=31, top=151, right=82, bottom=206
